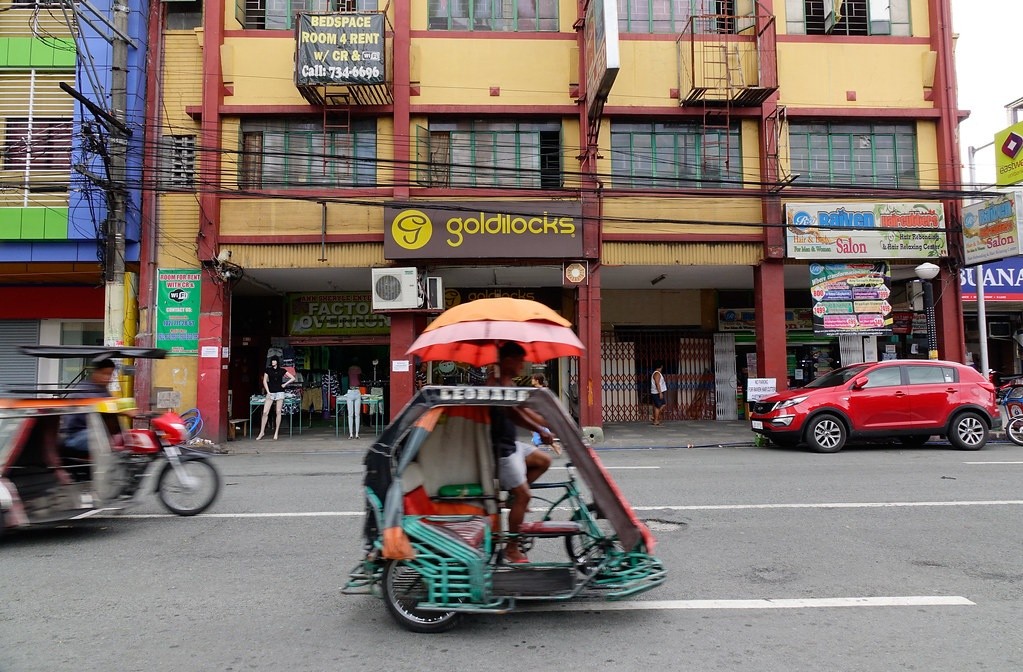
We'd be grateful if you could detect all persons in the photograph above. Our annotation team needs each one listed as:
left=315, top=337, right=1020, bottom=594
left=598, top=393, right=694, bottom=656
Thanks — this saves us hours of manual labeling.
left=650, top=361, right=667, bottom=424
left=346, top=387, right=361, bottom=439
left=529, top=374, right=563, bottom=457
left=487, top=341, right=557, bottom=565
left=414, top=362, right=428, bottom=391
left=256, top=355, right=295, bottom=440
left=57, top=358, right=122, bottom=451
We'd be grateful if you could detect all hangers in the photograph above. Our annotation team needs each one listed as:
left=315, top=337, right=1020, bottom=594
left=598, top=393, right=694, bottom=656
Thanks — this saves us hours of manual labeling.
left=359, top=381, right=389, bottom=388
left=307, top=381, right=321, bottom=389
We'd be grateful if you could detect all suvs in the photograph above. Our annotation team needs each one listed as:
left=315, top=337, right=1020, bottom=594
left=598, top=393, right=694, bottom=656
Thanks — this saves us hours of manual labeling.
left=748, top=359, right=1002, bottom=454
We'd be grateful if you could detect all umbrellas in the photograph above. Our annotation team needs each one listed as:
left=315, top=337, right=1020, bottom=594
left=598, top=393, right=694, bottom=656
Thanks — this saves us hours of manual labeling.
left=406, top=297, right=588, bottom=369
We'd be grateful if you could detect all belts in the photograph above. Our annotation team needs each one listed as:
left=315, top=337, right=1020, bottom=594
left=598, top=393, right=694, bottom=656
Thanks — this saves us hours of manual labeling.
left=348, top=386, right=359, bottom=390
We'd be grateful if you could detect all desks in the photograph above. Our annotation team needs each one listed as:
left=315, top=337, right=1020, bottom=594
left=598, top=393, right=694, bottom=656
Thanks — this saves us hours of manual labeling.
left=250, top=397, right=301, bottom=438
left=336, top=396, right=384, bottom=437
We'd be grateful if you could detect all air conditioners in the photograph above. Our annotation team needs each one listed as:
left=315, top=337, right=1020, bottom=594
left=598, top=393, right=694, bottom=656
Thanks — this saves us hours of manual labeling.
left=427, top=277, right=444, bottom=309
left=906, top=282, right=924, bottom=312
left=371, top=267, right=418, bottom=309
left=989, top=322, right=1011, bottom=338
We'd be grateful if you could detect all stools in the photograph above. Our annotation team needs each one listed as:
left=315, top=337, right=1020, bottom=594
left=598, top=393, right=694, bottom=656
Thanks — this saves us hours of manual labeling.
left=228, top=418, right=249, bottom=439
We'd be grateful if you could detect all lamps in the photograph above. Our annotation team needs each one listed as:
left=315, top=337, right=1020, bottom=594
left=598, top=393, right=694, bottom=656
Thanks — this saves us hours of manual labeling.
left=651, top=274, right=667, bottom=285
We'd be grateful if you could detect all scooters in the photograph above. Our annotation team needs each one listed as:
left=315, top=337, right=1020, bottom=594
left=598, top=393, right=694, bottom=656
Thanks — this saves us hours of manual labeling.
left=1005, top=413, right=1023, bottom=446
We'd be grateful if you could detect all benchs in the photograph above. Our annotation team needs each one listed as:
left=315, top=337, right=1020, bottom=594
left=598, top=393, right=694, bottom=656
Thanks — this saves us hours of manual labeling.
left=8, top=469, right=57, bottom=501
left=520, top=521, right=582, bottom=538
left=403, top=485, right=496, bottom=547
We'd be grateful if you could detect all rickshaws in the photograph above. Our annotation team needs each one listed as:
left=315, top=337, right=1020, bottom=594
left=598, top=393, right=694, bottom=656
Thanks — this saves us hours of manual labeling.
left=334, top=296, right=669, bottom=631
left=0, top=340, right=227, bottom=537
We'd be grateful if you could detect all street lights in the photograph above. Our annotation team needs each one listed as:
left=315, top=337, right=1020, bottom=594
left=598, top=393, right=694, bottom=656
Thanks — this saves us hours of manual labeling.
left=914, top=262, right=941, bottom=360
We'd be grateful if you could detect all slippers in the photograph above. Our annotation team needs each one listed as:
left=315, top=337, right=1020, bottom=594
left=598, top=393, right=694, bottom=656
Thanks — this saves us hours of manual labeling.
left=503, top=547, right=530, bottom=563
left=652, top=422, right=661, bottom=426
